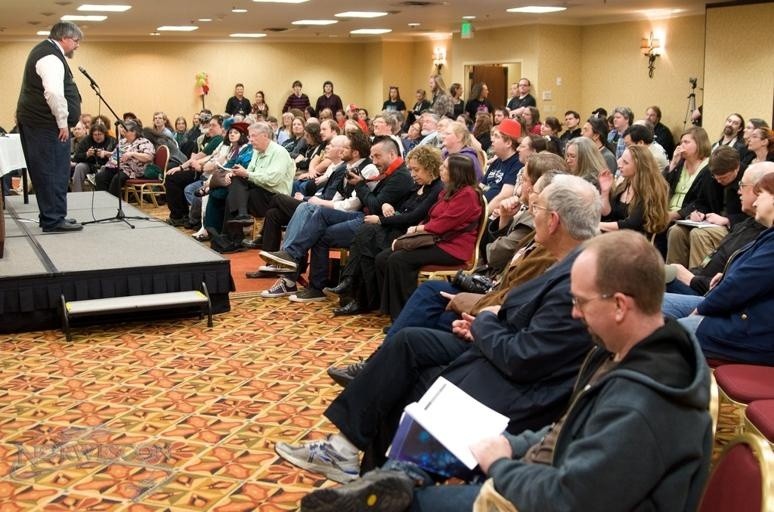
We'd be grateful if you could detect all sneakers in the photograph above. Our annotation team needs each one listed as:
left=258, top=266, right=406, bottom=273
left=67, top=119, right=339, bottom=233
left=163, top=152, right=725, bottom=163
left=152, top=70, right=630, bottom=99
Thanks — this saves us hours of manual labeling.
left=299, top=467, right=412, bottom=511
left=274, top=439, right=360, bottom=486
left=166, top=217, right=189, bottom=227
left=256, top=248, right=298, bottom=269
left=289, top=285, right=327, bottom=303
left=258, top=261, right=297, bottom=274
left=260, top=278, right=297, bottom=299
left=327, top=361, right=367, bottom=385
left=184, top=220, right=201, bottom=231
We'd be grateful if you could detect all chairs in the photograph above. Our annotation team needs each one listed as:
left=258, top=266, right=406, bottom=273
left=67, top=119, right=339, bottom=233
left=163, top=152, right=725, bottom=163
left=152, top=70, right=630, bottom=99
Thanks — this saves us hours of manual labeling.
left=413, top=195, right=487, bottom=307
left=697, top=432, right=774, bottom=511
left=710, top=368, right=719, bottom=432
left=714, top=364, right=773, bottom=399
left=125, top=145, right=172, bottom=208
left=745, top=398, right=774, bottom=446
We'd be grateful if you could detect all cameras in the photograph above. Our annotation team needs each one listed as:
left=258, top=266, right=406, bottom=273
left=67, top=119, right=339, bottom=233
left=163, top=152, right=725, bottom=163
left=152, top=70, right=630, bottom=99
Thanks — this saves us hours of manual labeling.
left=93, top=148, right=97, bottom=156
left=344, top=167, right=361, bottom=180
left=450, top=268, right=494, bottom=294
left=689, top=77, right=697, bottom=87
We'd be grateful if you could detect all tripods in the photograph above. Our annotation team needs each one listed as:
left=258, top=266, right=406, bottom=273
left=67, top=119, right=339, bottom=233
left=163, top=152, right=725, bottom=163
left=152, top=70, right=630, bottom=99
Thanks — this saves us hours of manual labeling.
left=79, top=86, right=149, bottom=230
left=681, top=85, right=699, bottom=131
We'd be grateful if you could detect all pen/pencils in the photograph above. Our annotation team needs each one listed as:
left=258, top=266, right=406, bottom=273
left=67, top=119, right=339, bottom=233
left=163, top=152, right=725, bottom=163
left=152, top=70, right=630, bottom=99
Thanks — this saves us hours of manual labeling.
left=692, top=205, right=703, bottom=222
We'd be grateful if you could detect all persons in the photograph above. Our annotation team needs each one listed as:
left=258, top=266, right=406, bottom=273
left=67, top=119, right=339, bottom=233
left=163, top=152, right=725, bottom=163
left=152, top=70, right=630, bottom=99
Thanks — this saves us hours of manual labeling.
left=297, top=231, right=715, bottom=511
left=16, top=22, right=85, bottom=233
left=276, top=173, right=604, bottom=485
left=72, top=74, right=774, bottom=362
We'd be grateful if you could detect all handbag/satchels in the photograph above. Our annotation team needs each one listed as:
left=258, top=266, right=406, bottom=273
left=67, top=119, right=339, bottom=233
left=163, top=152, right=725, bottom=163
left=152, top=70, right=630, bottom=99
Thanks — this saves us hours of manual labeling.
left=143, top=163, right=163, bottom=180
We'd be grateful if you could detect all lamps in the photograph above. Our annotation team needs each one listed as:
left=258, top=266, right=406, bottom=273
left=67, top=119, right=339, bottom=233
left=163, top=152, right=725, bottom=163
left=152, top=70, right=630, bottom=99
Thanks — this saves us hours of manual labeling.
left=641, top=28, right=665, bottom=79
left=433, top=46, right=447, bottom=72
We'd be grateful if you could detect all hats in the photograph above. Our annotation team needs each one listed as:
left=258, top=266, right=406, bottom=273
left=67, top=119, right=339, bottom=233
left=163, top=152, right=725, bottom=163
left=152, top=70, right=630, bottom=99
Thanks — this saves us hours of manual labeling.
left=495, top=118, right=523, bottom=140
left=592, top=108, right=607, bottom=117
left=345, top=104, right=358, bottom=111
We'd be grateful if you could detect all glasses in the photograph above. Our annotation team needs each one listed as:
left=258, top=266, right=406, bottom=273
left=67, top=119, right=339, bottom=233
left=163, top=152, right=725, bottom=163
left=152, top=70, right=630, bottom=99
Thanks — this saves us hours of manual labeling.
left=530, top=200, right=547, bottom=214
left=570, top=292, right=610, bottom=312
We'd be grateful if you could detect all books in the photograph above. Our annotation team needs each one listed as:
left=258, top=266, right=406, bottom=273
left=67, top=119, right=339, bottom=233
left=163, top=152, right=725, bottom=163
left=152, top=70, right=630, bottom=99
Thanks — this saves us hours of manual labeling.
left=407, top=375, right=513, bottom=470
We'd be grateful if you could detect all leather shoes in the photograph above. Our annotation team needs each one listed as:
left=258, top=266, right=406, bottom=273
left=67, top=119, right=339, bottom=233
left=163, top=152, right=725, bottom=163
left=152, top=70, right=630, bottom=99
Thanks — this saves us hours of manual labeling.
left=194, top=185, right=212, bottom=197
left=245, top=269, right=277, bottom=279
left=333, top=293, right=371, bottom=314
left=199, top=231, right=211, bottom=241
left=220, top=239, right=247, bottom=254
left=242, top=235, right=265, bottom=248
left=42, top=219, right=84, bottom=233
left=227, top=215, right=255, bottom=226
left=323, top=277, right=357, bottom=300
left=39, top=216, right=78, bottom=227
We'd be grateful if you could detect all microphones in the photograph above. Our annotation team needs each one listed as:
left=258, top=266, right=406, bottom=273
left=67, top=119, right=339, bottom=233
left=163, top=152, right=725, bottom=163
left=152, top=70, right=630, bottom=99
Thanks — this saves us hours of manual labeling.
left=78, top=66, right=96, bottom=86
